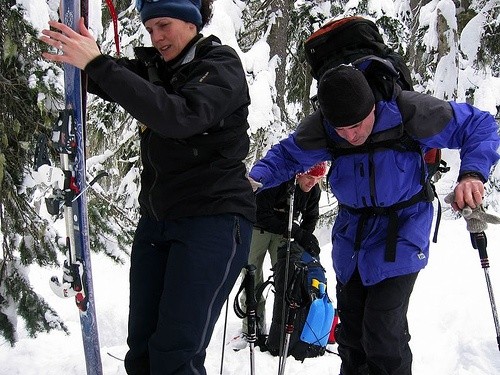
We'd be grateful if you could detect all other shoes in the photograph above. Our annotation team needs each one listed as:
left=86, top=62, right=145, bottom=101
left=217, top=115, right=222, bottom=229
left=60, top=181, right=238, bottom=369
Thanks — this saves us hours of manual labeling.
left=232, top=333, right=247, bottom=351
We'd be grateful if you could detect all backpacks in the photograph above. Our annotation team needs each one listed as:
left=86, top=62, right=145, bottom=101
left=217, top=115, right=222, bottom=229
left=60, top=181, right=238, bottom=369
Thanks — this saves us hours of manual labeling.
left=264, top=240, right=335, bottom=363
left=304, top=18, right=442, bottom=182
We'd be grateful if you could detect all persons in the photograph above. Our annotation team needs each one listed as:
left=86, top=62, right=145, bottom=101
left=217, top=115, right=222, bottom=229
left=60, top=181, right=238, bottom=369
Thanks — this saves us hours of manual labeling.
left=244, top=65, right=500, bottom=375
left=233, top=160, right=327, bottom=350
left=40, top=0, right=257, bottom=375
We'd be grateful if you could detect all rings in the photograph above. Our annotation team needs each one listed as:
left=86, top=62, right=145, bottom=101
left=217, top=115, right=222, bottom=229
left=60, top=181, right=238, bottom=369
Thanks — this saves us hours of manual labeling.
left=474, top=191, right=479, bottom=193
left=59, top=44, right=64, bottom=49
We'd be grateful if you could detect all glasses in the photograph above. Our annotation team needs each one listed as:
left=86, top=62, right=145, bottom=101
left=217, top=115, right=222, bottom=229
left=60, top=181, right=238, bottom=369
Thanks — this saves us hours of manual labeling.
left=135, top=0, right=159, bottom=12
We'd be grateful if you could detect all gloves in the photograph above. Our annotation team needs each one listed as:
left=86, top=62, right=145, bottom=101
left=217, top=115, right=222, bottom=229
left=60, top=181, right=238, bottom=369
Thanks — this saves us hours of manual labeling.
left=444, top=191, right=500, bottom=233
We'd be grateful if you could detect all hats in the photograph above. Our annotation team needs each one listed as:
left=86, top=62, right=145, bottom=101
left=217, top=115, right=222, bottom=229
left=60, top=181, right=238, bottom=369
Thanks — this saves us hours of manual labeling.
left=318, top=65, right=376, bottom=127
left=141, top=0, right=203, bottom=26
left=304, top=161, right=326, bottom=176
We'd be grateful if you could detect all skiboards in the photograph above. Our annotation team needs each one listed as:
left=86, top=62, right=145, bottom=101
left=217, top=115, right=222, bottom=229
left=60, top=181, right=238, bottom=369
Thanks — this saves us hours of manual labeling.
left=58, top=0, right=103, bottom=375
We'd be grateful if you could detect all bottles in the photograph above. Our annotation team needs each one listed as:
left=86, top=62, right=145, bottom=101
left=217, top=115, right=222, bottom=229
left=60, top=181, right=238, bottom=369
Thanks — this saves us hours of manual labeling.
left=327, top=308, right=339, bottom=344
